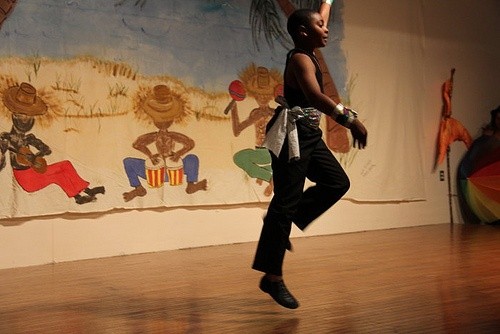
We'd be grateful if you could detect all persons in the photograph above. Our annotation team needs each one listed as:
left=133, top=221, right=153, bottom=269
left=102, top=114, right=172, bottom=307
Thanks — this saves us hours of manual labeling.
left=251, top=0, right=369, bottom=311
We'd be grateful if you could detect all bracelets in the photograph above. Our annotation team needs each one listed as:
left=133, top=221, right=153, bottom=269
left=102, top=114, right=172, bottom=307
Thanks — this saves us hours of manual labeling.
left=323, top=0, right=333, bottom=5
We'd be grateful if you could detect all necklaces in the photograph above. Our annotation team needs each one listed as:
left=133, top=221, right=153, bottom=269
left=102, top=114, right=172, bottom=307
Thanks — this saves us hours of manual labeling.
left=305, top=50, right=321, bottom=71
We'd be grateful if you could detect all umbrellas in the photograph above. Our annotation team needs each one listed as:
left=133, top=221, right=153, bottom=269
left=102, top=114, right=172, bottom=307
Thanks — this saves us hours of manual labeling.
left=456, top=135, right=500, bottom=226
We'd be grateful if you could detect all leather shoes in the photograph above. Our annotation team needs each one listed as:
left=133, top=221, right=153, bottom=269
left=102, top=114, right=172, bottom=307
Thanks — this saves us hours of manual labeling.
left=259, top=275, right=298, bottom=308
left=263, top=216, right=292, bottom=251
